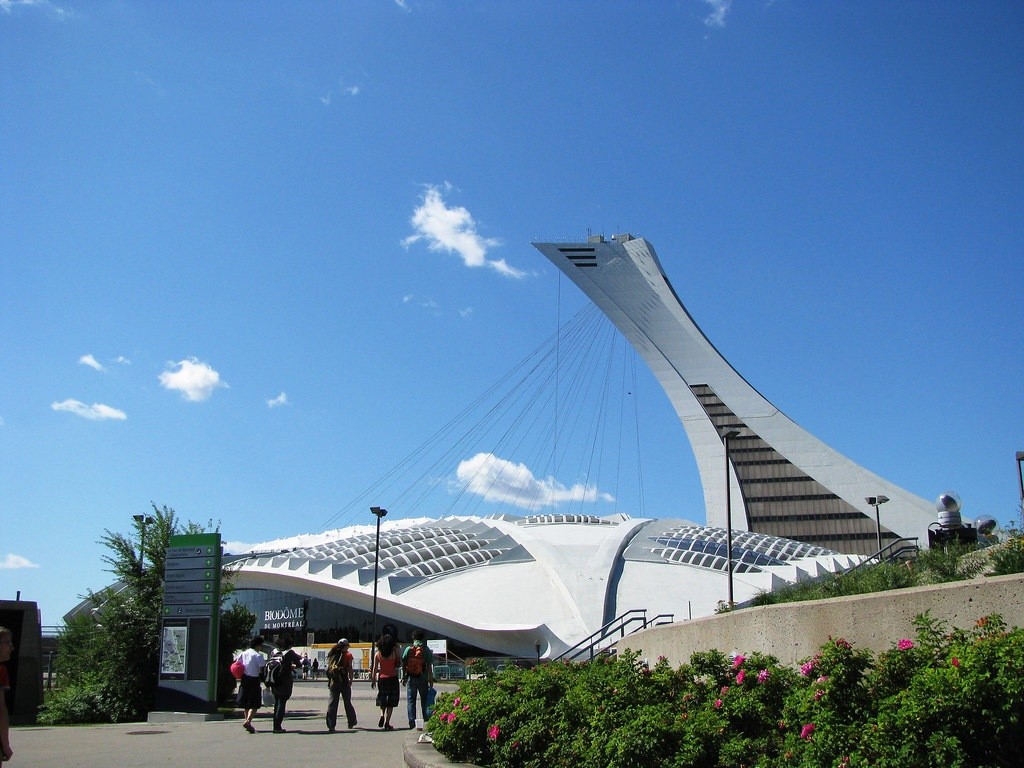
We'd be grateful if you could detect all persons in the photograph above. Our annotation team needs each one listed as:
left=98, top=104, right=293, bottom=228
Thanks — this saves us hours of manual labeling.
left=272, top=650, right=302, bottom=733
left=311, top=658, right=319, bottom=679
left=326, top=638, right=357, bottom=731
left=301, top=656, right=309, bottom=679
left=371, top=634, right=402, bottom=729
left=400, top=629, right=433, bottom=729
left=0, top=627, right=15, bottom=767
left=235, top=636, right=265, bottom=733
left=308, top=659, right=312, bottom=676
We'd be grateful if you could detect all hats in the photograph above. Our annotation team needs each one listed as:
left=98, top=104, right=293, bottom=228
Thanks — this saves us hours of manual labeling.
left=338, top=637, right=349, bottom=646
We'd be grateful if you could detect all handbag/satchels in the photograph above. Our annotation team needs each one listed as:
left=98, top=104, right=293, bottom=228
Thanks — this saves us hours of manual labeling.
left=394, top=648, right=401, bottom=668
left=230, top=658, right=244, bottom=679
left=425, top=686, right=437, bottom=716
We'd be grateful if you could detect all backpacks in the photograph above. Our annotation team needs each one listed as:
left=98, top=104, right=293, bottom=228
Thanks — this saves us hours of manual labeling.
left=275, top=658, right=294, bottom=701
left=406, top=645, right=426, bottom=676
left=265, top=659, right=280, bottom=688
left=326, top=652, right=346, bottom=689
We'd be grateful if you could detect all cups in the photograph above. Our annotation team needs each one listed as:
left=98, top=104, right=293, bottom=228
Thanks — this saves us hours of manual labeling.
left=415, top=718, right=424, bottom=731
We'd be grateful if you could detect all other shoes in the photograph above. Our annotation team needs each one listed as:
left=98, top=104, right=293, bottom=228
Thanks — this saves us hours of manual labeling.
left=348, top=720, right=357, bottom=729
left=424, top=718, right=429, bottom=722
left=329, top=728, right=335, bottom=731
left=409, top=720, right=415, bottom=729
left=243, top=722, right=255, bottom=733
left=273, top=721, right=286, bottom=732
left=378, top=716, right=384, bottom=727
left=385, top=723, right=393, bottom=729
